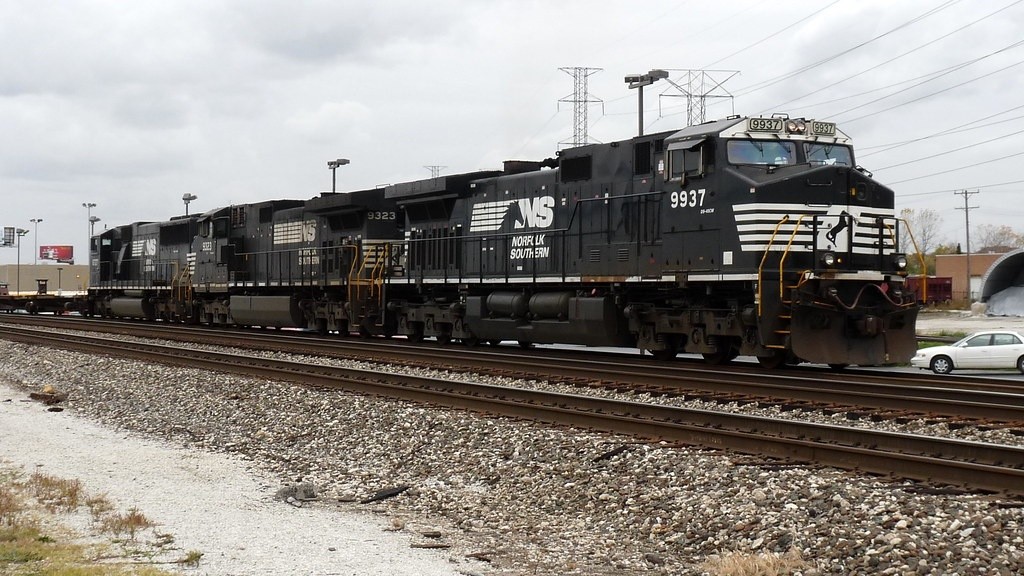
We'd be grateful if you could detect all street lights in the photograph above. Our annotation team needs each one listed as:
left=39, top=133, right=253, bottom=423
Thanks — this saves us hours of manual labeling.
left=30, top=219, right=43, bottom=264
left=56, top=266, right=63, bottom=289
left=327, top=159, right=350, bottom=194
left=89, top=216, right=101, bottom=237
left=82, top=202, right=97, bottom=265
left=624, top=69, right=671, bottom=138
left=182, top=193, right=196, bottom=217
left=15, top=228, right=32, bottom=295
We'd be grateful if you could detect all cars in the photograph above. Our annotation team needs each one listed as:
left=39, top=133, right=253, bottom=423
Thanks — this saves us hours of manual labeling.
left=911, top=330, right=1024, bottom=375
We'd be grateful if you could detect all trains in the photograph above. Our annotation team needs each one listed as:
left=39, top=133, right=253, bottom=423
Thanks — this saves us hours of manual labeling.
left=86, top=111, right=927, bottom=371
